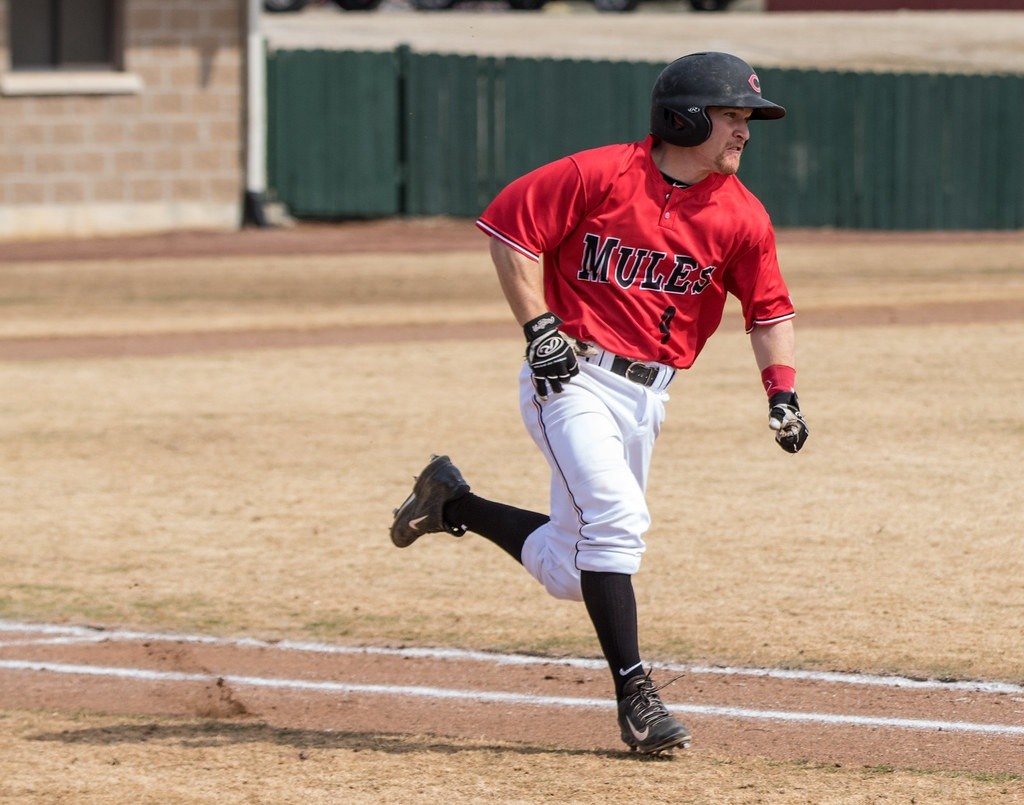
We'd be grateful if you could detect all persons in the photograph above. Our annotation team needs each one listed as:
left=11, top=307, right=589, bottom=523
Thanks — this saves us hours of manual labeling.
left=388, top=50, right=807, bottom=754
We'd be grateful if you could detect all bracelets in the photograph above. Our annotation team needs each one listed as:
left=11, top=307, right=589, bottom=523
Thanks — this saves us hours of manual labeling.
left=762, top=364, right=796, bottom=395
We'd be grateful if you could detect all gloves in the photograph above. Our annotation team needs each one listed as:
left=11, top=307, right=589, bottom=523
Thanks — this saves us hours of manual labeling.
left=523, top=311, right=599, bottom=402
left=768, top=393, right=809, bottom=453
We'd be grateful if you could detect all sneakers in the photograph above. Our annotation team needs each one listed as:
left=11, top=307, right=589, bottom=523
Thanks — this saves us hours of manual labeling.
left=390, top=454, right=470, bottom=548
left=619, top=675, right=691, bottom=758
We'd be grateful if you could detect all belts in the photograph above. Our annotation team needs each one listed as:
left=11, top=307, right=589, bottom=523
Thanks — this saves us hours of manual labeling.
left=610, top=355, right=676, bottom=389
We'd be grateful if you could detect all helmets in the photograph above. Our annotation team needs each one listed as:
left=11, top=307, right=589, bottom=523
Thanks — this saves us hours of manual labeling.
left=651, top=52, right=786, bottom=147
